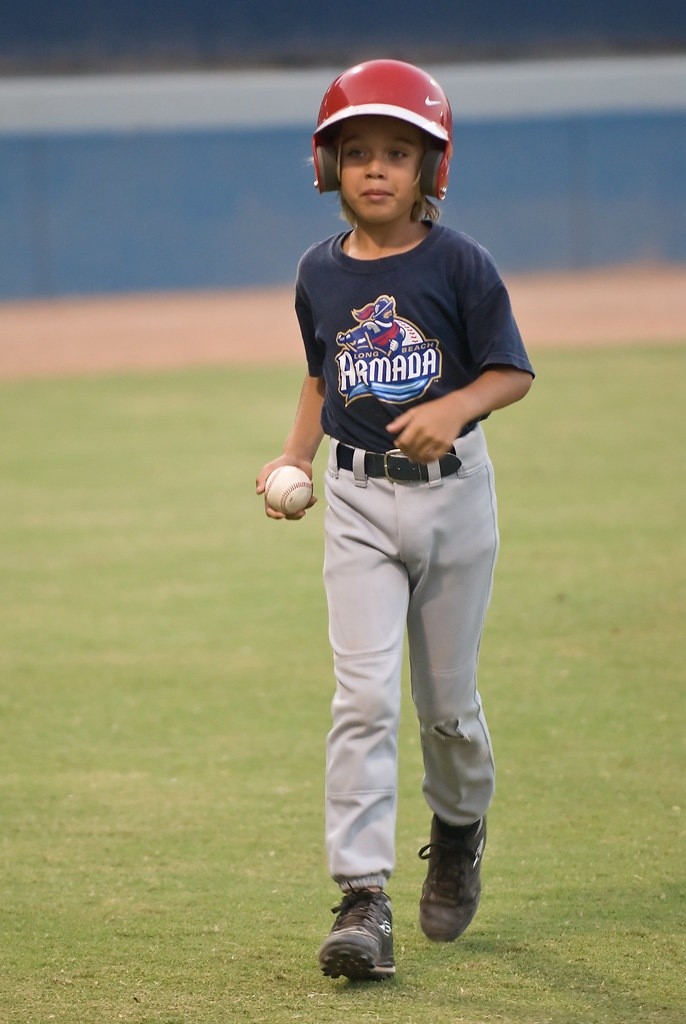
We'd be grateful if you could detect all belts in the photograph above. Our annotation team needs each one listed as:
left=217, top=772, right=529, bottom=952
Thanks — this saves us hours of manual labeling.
left=337, top=446, right=463, bottom=483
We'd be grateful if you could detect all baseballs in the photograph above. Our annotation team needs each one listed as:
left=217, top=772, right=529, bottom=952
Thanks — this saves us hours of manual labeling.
left=265, top=464, right=313, bottom=516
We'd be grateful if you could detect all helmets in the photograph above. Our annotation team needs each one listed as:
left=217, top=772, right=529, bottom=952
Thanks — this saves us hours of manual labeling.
left=312, top=59, right=453, bottom=199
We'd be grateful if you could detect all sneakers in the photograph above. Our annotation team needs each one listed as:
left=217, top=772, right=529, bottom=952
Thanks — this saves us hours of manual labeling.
left=419, top=813, right=487, bottom=942
left=316, top=880, right=397, bottom=980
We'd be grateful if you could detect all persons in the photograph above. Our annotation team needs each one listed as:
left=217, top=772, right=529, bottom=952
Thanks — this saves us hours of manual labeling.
left=255, top=58, right=536, bottom=980
